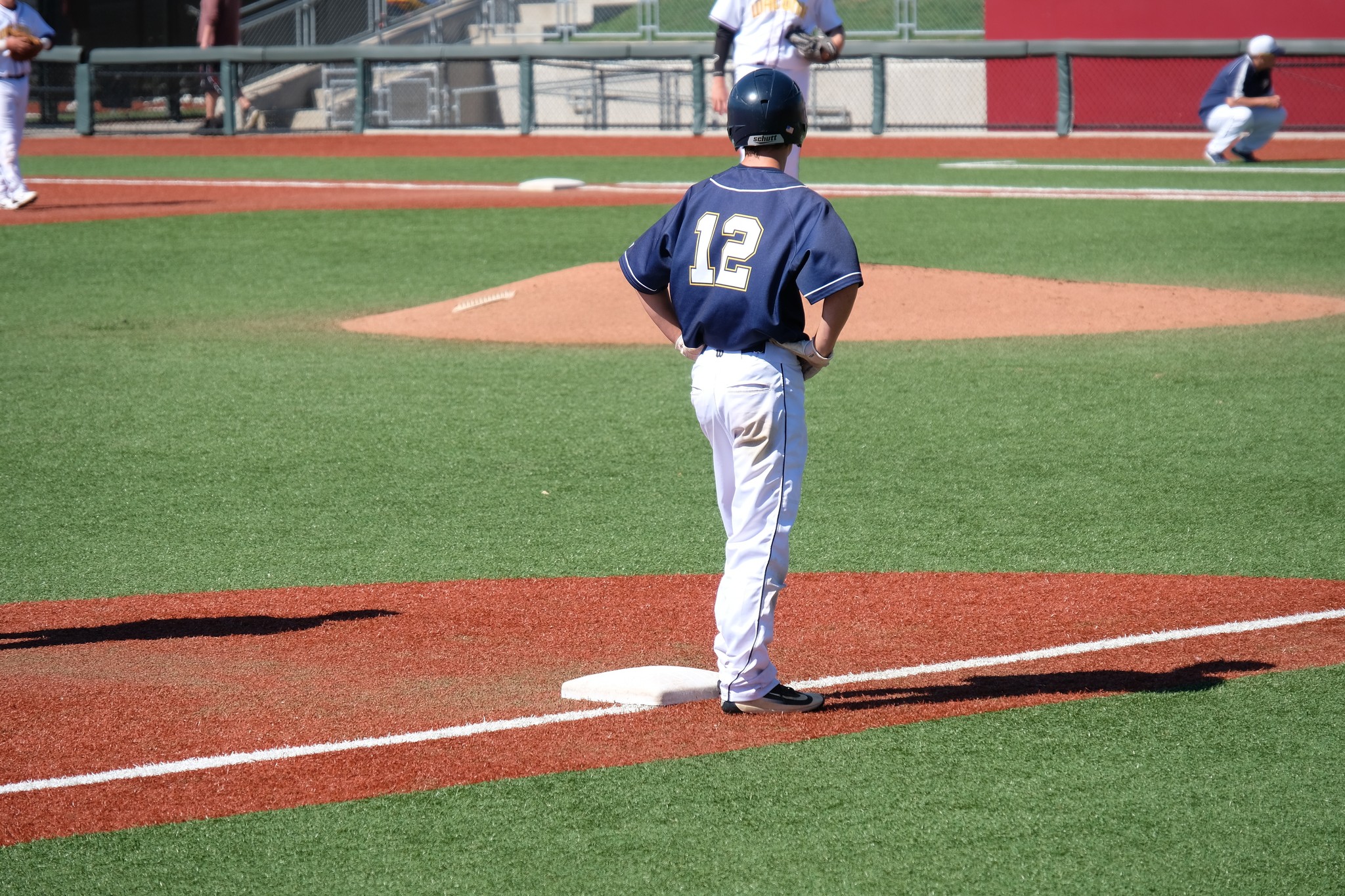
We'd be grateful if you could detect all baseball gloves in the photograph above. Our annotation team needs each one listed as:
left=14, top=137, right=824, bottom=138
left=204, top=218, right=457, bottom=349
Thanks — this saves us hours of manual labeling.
left=4, top=27, right=43, bottom=62
left=787, top=27, right=839, bottom=65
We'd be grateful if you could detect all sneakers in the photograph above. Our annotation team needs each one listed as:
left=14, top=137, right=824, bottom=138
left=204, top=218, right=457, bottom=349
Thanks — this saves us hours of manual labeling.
left=717, top=678, right=825, bottom=714
left=1206, top=149, right=1231, bottom=163
left=12, top=187, right=38, bottom=207
left=0, top=195, right=23, bottom=210
left=1231, top=146, right=1260, bottom=162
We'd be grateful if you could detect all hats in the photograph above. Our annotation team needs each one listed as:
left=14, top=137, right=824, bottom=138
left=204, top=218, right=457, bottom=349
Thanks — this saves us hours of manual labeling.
left=1247, top=34, right=1286, bottom=56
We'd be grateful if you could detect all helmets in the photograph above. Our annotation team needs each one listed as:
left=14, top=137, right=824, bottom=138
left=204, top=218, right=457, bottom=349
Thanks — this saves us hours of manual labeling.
left=728, top=67, right=809, bottom=149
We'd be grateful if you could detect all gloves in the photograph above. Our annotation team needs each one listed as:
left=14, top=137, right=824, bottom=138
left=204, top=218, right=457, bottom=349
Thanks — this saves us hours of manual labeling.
left=772, top=337, right=834, bottom=380
left=674, top=333, right=701, bottom=361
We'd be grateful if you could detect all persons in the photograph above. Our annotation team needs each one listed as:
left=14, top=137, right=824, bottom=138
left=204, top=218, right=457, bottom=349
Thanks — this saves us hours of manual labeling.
left=189, top=0, right=259, bottom=131
left=0, top=0, right=57, bottom=211
left=1198, top=35, right=1288, bottom=166
left=616, top=68, right=866, bottom=714
left=706, top=0, right=845, bottom=181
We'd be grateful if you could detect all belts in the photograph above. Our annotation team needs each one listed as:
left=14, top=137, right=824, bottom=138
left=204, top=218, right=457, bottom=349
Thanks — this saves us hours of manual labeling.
left=9, top=74, right=26, bottom=80
left=699, top=342, right=768, bottom=356
